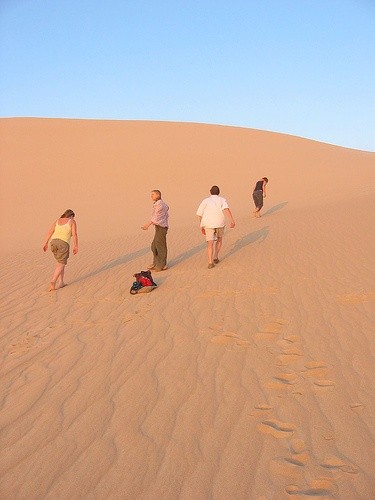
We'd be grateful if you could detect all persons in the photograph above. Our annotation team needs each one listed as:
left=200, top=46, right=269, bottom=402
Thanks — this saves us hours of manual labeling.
left=196, top=185, right=234, bottom=269
left=140, top=189, right=169, bottom=272
left=252, top=176, right=268, bottom=217
left=42, top=208, right=82, bottom=289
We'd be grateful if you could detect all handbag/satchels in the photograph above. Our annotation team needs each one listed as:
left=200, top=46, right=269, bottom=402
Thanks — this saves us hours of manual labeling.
left=130, top=270, right=158, bottom=295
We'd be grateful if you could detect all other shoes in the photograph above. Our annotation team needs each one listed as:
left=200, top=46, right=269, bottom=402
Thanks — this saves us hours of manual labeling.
left=208, top=264, right=215, bottom=269
left=213, top=258, right=220, bottom=264
left=148, top=264, right=167, bottom=271
